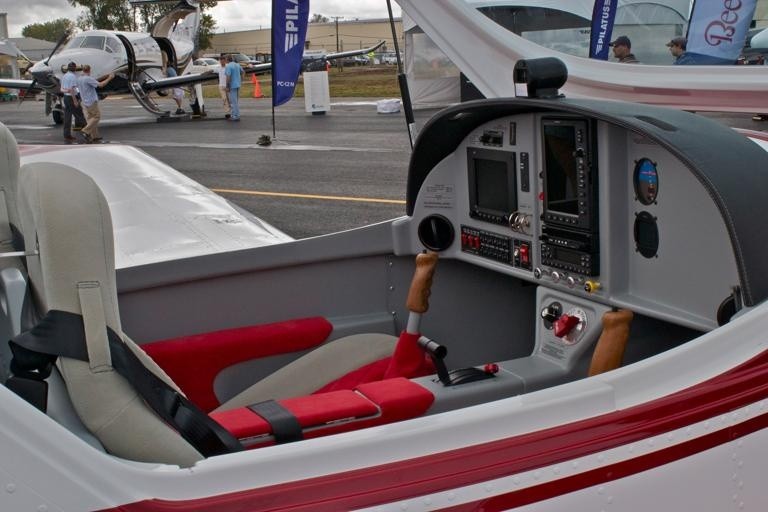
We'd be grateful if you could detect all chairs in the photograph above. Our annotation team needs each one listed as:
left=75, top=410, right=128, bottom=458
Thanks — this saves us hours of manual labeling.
left=2, top=123, right=435, bottom=468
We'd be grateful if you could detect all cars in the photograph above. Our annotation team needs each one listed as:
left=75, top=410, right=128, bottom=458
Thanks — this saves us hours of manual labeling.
left=190, top=48, right=402, bottom=72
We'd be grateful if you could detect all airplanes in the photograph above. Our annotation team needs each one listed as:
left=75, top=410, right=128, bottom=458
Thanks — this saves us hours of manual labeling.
left=1, top=0, right=385, bottom=124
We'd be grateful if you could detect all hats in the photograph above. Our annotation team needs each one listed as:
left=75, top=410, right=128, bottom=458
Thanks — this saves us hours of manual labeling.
left=609, top=36, right=631, bottom=49
left=666, top=38, right=686, bottom=46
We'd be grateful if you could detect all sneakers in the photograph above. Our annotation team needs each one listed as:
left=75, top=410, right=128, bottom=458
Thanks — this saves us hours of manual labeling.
left=225, top=112, right=240, bottom=121
left=64, top=135, right=77, bottom=141
left=73, top=126, right=82, bottom=131
left=80, top=131, right=92, bottom=144
left=94, top=138, right=109, bottom=144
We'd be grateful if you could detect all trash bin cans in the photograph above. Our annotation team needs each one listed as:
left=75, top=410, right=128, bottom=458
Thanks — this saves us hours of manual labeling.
left=303, top=61, right=330, bottom=115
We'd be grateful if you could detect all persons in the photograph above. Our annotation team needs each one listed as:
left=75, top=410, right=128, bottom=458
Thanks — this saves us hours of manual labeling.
left=609, top=35, right=640, bottom=63
left=368, top=52, right=375, bottom=68
left=225, top=57, right=245, bottom=121
left=167, top=67, right=185, bottom=114
left=60, top=62, right=87, bottom=140
left=78, top=65, right=115, bottom=141
left=665, top=37, right=696, bottom=64
left=212, top=57, right=231, bottom=119
left=336, top=58, right=343, bottom=72
